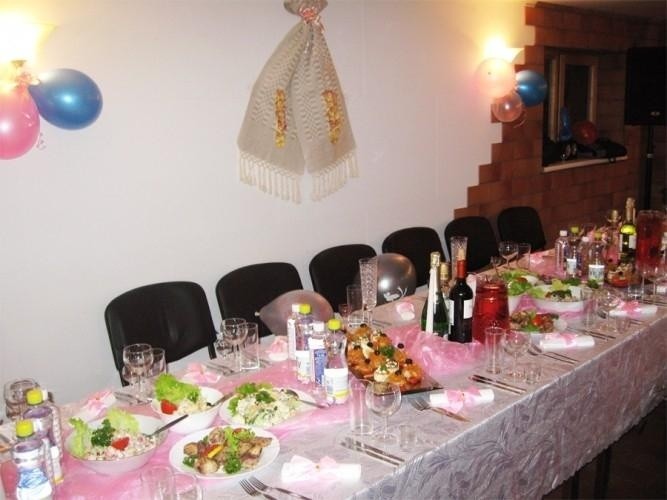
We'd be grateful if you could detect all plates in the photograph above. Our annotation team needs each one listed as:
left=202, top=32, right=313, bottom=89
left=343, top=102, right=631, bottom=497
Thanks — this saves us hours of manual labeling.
left=220, top=386, right=316, bottom=427
left=168, top=423, right=279, bottom=478
left=527, top=283, right=594, bottom=313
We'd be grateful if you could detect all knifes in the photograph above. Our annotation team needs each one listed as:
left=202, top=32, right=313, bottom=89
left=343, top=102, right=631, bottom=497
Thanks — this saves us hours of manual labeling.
left=352, top=315, right=392, bottom=329
left=568, top=328, right=616, bottom=342
left=340, top=441, right=399, bottom=467
left=345, top=438, right=405, bottom=461
left=469, top=376, right=520, bottom=396
left=472, top=374, right=527, bottom=391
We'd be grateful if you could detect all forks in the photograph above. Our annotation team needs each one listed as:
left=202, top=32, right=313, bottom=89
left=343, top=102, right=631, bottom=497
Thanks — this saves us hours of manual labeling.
left=530, top=344, right=580, bottom=361
left=238, top=479, right=277, bottom=500
left=410, top=397, right=459, bottom=418
left=417, top=394, right=466, bottom=422
left=114, top=391, right=153, bottom=405
left=527, top=349, right=574, bottom=365
left=245, top=352, right=271, bottom=369
left=249, top=476, right=313, bottom=500
left=209, top=361, right=235, bottom=377
left=115, top=396, right=143, bottom=408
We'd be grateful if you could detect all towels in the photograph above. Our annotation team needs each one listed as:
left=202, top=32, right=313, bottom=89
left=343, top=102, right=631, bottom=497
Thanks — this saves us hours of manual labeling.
left=526, top=252, right=543, bottom=264
left=607, top=302, right=658, bottom=317
left=69, top=391, right=115, bottom=427
left=537, top=331, right=594, bottom=351
left=396, top=301, right=414, bottom=321
left=178, top=364, right=221, bottom=384
left=466, top=274, right=484, bottom=297
left=268, top=336, right=288, bottom=360
left=282, top=454, right=362, bottom=487
left=656, top=283, right=666, bottom=294
left=430, top=388, right=495, bottom=407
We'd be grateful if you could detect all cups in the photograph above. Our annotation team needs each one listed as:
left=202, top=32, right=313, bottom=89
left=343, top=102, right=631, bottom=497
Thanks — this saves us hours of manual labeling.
left=499, top=240, right=518, bottom=271
left=350, top=379, right=374, bottom=437
left=485, top=326, right=505, bottom=375
left=238, top=323, right=259, bottom=371
left=358, top=259, right=379, bottom=331
left=348, top=286, right=364, bottom=327
left=588, top=263, right=606, bottom=286
left=518, top=244, right=531, bottom=273
left=365, top=379, right=402, bottom=445
left=340, top=304, right=352, bottom=328
left=121, top=366, right=138, bottom=394
left=628, top=266, right=644, bottom=299
left=565, top=258, right=577, bottom=277
left=449, top=235, right=468, bottom=296
left=501, top=326, right=531, bottom=381
left=161, top=473, right=199, bottom=500
left=490, top=257, right=503, bottom=280
left=3, top=378, right=34, bottom=417
left=139, top=466, right=177, bottom=500
left=220, top=318, right=248, bottom=371
left=214, top=342, right=233, bottom=368
left=123, top=343, right=155, bottom=399
left=596, top=287, right=618, bottom=332
left=143, top=349, right=167, bottom=385
left=616, top=311, right=631, bottom=333
left=643, top=265, right=665, bottom=300
left=581, top=288, right=596, bottom=328
left=526, top=358, right=543, bottom=386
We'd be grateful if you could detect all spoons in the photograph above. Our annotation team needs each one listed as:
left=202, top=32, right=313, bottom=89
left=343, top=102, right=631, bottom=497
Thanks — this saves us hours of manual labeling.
left=142, top=414, right=188, bottom=441
left=280, top=387, right=326, bottom=408
left=206, top=394, right=233, bottom=411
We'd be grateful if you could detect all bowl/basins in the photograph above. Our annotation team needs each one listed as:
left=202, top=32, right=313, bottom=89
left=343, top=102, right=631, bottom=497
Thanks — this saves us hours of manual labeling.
left=152, top=386, right=223, bottom=435
left=64, top=411, right=165, bottom=477
left=495, top=269, right=538, bottom=315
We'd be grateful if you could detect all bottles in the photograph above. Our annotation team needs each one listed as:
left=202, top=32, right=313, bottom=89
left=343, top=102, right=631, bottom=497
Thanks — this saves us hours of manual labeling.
left=554, top=230, right=567, bottom=273
left=286, top=304, right=296, bottom=361
left=565, top=227, right=581, bottom=282
left=293, top=304, right=315, bottom=384
left=617, top=198, right=637, bottom=264
left=587, top=232, right=604, bottom=290
left=438, top=262, right=453, bottom=337
left=23, top=390, right=64, bottom=486
left=447, top=248, right=473, bottom=344
left=420, top=252, right=448, bottom=338
left=322, top=319, right=352, bottom=405
left=10, top=421, right=52, bottom=500
left=36, top=389, right=64, bottom=462
left=307, top=321, right=326, bottom=391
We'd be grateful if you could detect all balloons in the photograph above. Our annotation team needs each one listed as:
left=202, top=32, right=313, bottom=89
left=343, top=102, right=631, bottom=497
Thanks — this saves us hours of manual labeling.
left=569, top=120, right=597, bottom=145
left=512, top=70, right=548, bottom=106
left=490, top=90, right=523, bottom=122
left=26, top=67, right=103, bottom=131
left=475, top=57, right=515, bottom=98
left=0, top=64, right=40, bottom=158
left=559, top=107, right=580, bottom=139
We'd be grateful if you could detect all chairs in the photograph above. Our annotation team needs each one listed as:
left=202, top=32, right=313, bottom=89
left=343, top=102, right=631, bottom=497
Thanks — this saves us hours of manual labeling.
left=105, top=282, right=218, bottom=390
left=443, top=215, right=496, bottom=280
left=381, top=225, right=446, bottom=288
left=215, top=262, right=304, bottom=352
left=309, top=240, right=377, bottom=311
left=496, top=206, right=547, bottom=266
